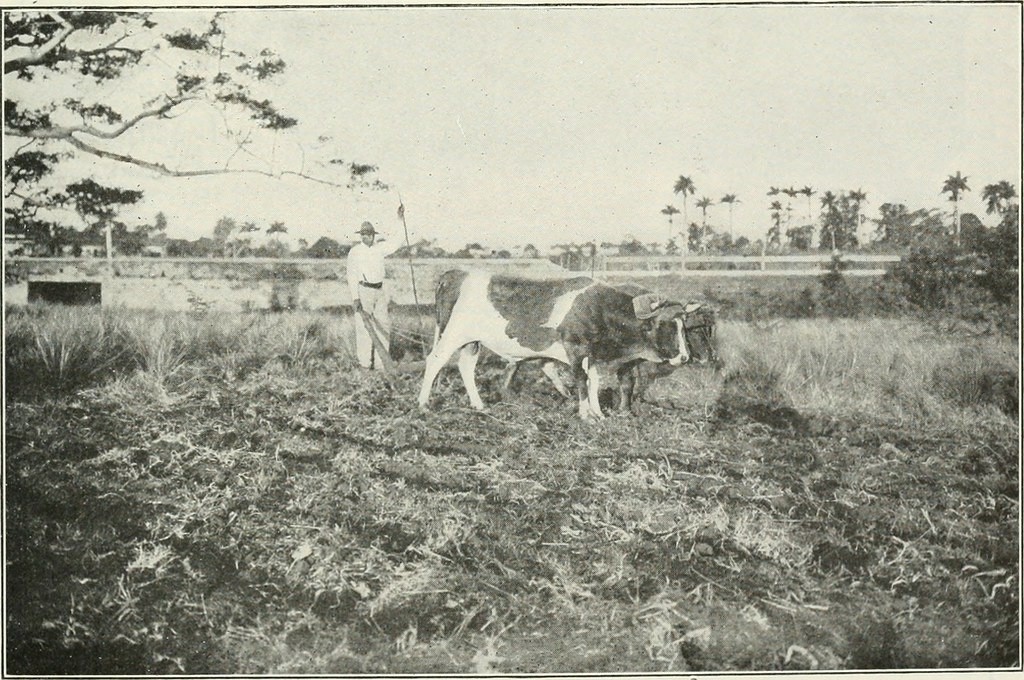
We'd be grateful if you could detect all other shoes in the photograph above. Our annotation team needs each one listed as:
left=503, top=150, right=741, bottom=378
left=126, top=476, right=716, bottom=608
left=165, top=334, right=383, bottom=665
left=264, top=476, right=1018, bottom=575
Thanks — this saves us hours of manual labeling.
left=359, top=361, right=371, bottom=369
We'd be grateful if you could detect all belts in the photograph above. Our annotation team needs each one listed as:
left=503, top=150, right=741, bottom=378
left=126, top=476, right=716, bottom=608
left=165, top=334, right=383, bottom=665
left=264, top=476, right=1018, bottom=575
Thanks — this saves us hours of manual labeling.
left=359, top=280, right=383, bottom=289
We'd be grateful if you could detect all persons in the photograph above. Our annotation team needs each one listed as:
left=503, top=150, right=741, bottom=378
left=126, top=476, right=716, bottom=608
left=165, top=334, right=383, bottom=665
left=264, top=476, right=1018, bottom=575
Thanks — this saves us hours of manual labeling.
left=346, top=204, right=405, bottom=370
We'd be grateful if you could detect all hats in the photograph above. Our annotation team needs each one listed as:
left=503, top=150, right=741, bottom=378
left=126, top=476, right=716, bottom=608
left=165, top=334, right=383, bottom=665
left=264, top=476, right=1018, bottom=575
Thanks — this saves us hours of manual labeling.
left=355, top=221, right=379, bottom=234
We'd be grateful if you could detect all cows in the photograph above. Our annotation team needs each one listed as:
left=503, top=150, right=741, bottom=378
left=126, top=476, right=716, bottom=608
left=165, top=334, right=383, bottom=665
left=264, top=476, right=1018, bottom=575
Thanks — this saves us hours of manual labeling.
left=419, top=269, right=719, bottom=424
